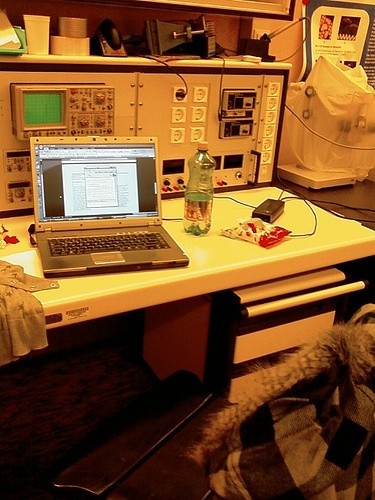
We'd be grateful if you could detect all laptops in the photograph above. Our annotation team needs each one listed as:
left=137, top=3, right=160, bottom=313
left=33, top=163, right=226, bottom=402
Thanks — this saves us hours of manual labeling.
left=30, top=136, right=189, bottom=278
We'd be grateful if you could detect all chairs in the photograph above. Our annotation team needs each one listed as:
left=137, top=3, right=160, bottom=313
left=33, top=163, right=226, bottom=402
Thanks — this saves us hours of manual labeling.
left=20, top=304, right=374, bottom=494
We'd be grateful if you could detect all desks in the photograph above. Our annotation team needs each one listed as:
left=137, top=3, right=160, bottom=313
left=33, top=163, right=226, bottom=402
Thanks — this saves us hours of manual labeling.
left=0, top=187, right=375, bottom=397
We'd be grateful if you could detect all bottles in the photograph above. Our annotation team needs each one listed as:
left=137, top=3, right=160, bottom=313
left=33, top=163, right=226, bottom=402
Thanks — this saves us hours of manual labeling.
left=183, top=142, right=217, bottom=235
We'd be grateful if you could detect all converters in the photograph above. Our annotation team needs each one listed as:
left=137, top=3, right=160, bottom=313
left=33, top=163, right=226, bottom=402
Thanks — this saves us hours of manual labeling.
left=252, top=199, right=285, bottom=224
